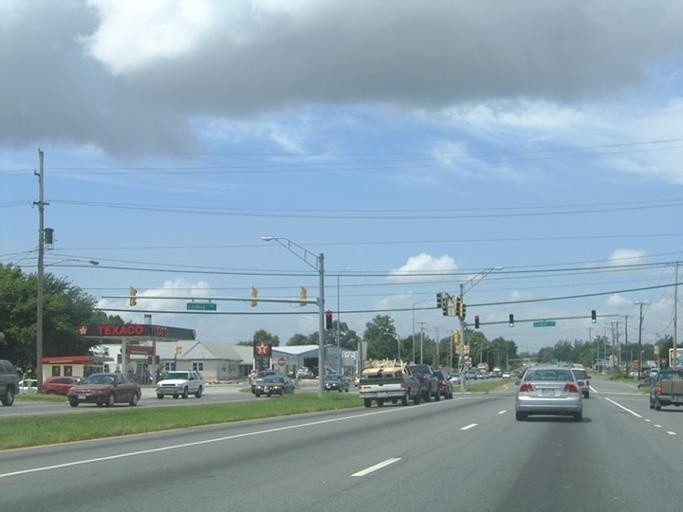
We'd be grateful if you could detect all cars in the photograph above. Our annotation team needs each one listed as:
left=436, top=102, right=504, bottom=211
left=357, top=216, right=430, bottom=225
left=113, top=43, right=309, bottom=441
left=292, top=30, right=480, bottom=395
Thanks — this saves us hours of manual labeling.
left=517, top=368, right=591, bottom=421
left=20, top=379, right=45, bottom=393
left=360, top=364, right=511, bottom=408
left=156, top=370, right=206, bottom=399
left=324, top=374, right=350, bottom=392
left=248, top=369, right=295, bottom=399
left=649, top=368, right=683, bottom=410
left=0, top=359, right=20, bottom=406
left=68, top=372, right=141, bottom=407
left=45, top=376, right=82, bottom=395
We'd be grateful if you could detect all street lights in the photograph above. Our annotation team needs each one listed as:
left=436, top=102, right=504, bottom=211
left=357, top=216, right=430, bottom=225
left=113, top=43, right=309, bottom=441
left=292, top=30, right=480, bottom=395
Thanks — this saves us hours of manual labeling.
left=260, top=236, right=324, bottom=394
left=458, top=266, right=503, bottom=390
left=44, top=259, right=99, bottom=267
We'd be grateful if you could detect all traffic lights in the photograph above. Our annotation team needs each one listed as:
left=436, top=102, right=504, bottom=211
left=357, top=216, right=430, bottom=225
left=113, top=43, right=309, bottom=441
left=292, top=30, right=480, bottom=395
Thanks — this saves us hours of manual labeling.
left=592, top=310, right=597, bottom=324
left=454, top=329, right=470, bottom=355
left=436, top=290, right=467, bottom=320
left=509, top=314, right=514, bottom=327
left=474, top=316, right=480, bottom=328
left=326, top=310, right=333, bottom=329
